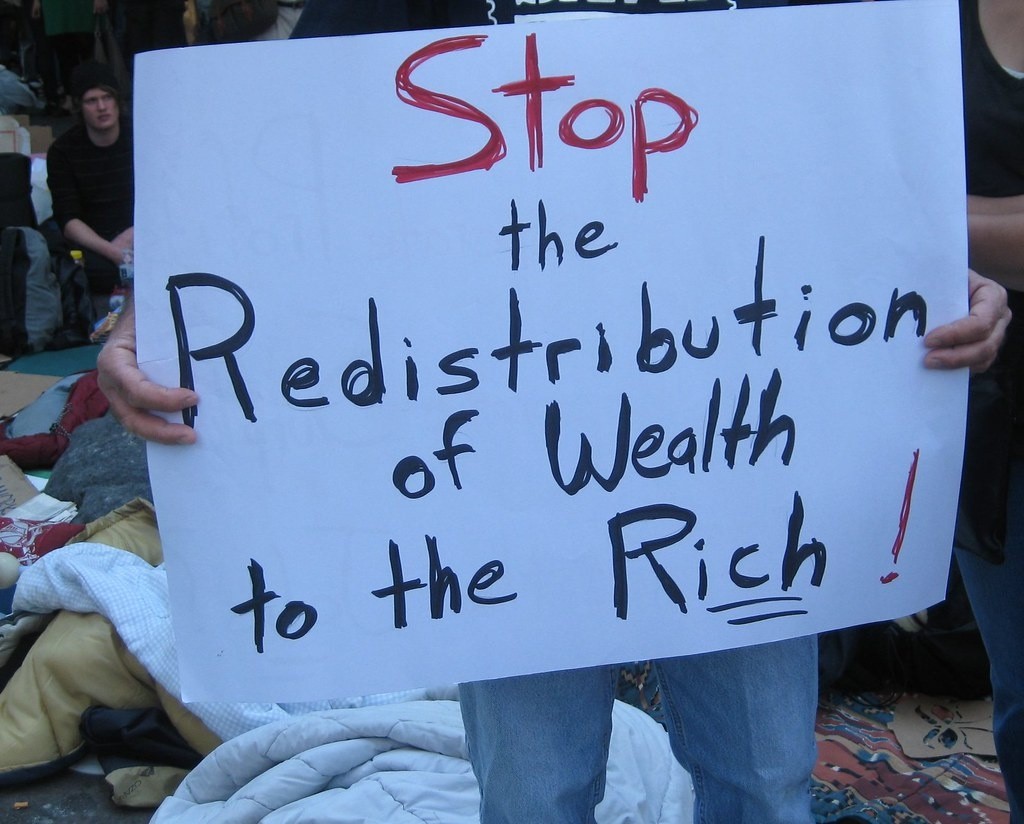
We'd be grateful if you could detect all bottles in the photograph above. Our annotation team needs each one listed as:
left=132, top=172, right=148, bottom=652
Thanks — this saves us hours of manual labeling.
left=120, top=247, right=135, bottom=289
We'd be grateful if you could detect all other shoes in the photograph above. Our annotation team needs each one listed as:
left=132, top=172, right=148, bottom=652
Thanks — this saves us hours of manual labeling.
left=88, top=299, right=112, bottom=343
left=53, top=96, right=77, bottom=116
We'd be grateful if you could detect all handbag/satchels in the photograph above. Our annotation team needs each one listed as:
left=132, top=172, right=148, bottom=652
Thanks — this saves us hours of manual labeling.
left=210, top=0, right=278, bottom=44
left=95, top=12, right=125, bottom=74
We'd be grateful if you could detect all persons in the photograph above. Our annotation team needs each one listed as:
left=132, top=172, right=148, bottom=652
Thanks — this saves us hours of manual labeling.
left=953, top=0, right=1024, bottom=824
left=94, top=1, right=1015, bottom=824
left=3, top=1, right=307, bottom=295
